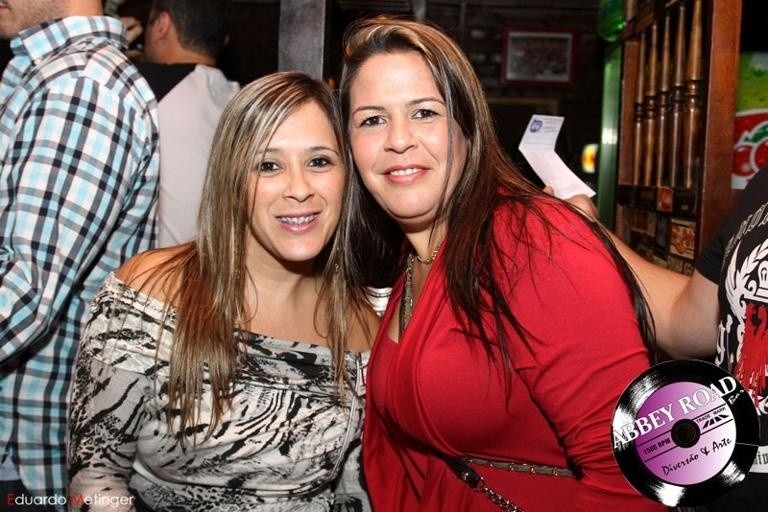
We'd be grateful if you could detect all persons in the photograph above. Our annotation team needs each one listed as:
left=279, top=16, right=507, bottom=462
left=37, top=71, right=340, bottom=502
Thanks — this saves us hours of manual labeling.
left=336, top=17, right=687, bottom=512
left=0, top=0, right=163, bottom=512
left=63, top=66, right=385, bottom=511
left=127, top=0, right=247, bottom=254
left=538, top=154, right=768, bottom=511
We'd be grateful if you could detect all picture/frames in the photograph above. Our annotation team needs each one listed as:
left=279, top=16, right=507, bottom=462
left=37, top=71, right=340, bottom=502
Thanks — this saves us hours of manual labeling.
left=501, top=26, right=578, bottom=88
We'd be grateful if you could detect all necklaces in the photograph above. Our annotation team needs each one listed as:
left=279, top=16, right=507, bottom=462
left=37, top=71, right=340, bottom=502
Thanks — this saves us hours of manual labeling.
left=410, top=245, right=440, bottom=265
